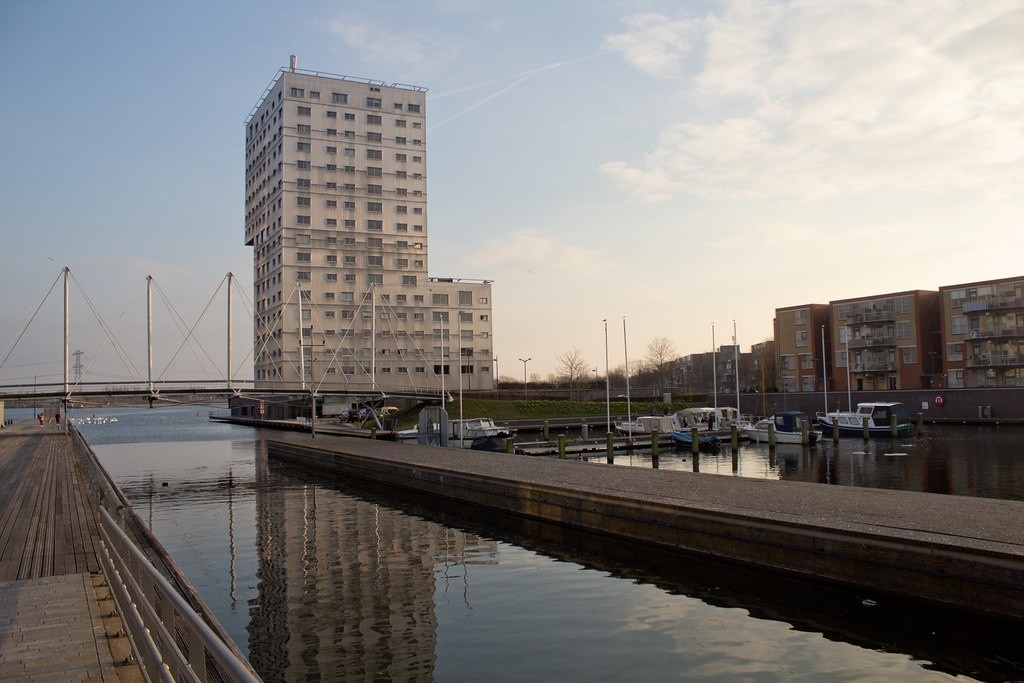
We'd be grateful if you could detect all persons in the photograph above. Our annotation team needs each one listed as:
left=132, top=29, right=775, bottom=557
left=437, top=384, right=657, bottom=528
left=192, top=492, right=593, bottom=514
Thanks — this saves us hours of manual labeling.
left=39, top=413, right=44, bottom=428
left=707, top=414, right=713, bottom=431
left=684, top=416, right=708, bottom=428
left=55, top=411, right=61, bottom=425
left=346, top=412, right=353, bottom=423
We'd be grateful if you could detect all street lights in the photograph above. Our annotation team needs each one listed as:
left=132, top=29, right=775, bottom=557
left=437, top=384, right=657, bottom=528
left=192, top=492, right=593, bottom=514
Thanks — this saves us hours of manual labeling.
left=518, top=357, right=532, bottom=400
left=593, top=366, right=598, bottom=386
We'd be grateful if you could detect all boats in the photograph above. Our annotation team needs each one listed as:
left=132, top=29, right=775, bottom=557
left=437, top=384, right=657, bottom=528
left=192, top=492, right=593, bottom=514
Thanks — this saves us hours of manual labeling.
left=394, top=417, right=519, bottom=452
left=672, top=431, right=720, bottom=448
left=674, top=407, right=724, bottom=431
left=614, top=416, right=676, bottom=437
left=815, top=322, right=915, bottom=436
left=744, top=410, right=822, bottom=444
